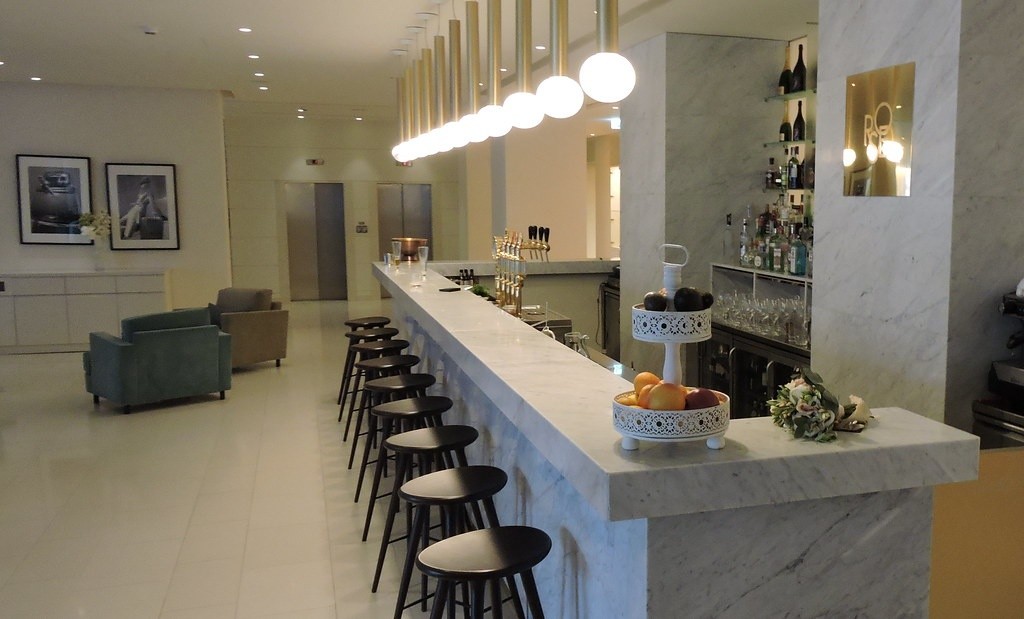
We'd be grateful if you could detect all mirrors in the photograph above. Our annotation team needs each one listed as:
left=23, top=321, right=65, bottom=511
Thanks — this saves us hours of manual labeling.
left=843, top=61, right=916, bottom=197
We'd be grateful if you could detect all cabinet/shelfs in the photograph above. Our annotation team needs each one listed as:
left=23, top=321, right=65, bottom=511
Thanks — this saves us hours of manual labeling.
left=697, top=85, right=818, bottom=419
left=599, top=283, right=620, bottom=364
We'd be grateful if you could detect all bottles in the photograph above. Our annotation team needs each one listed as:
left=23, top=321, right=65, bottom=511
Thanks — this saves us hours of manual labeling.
left=793, top=101, right=805, bottom=142
left=723, top=145, right=813, bottom=279
left=791, top=44, right=806, bottom=93
left=779, top=99, right=791, bottom=142
left=459, top=268, right=475, bottom=285
left=777, top=47, right=792, bottom=96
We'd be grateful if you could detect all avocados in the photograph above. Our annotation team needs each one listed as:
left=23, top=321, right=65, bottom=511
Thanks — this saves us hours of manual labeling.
left=643, top=286, right=713, bottom=312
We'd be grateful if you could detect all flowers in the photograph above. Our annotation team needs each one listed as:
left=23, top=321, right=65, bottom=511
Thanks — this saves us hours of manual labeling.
left=78, top=207, right=112, bottom=241
left=763, top=365, right=875, bottom=444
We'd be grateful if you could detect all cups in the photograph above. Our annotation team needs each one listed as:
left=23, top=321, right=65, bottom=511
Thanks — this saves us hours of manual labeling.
left=392, top=241, right=401, bottom=273
left=418, top=246, right=428, bottom=277
left=715, top=290, right=808, bottom=347
left=384, top=253, right=392, bottom=264
left=408, top=272, right=426, bottom=286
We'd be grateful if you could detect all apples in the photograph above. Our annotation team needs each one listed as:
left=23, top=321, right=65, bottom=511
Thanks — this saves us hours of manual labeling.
left=645, top=382, right=720, bottom=411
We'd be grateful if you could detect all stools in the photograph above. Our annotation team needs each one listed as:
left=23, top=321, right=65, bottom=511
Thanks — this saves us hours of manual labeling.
left=337, top=317, right=392, bottom=406
left=338, top=326, right=406, bottom=427
left=343, top=339, right=416, bottom=449
left=347, top=355, right=433, bottom=478
left=354, top=373, right=451, bottom=513
left=415, top=526, right=552, bottom=619
left=394, top=465, right=527, bottom=619
left=362, top=395, right=475, bottom=543
left=371, top=425, right=486, bottom=593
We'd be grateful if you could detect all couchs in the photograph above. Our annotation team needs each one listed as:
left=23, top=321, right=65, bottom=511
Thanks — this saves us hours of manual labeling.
left=82, top=307, right=231, bottom=414
left=173, top=287, right=288, bottom=374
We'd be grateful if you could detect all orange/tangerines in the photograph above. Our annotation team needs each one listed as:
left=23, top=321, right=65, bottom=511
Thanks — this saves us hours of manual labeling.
left=618, top=371, right=662, bottom=411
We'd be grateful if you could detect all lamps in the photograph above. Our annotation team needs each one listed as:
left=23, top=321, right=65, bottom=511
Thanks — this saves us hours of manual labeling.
left=392, top=0, right=636, bottom=177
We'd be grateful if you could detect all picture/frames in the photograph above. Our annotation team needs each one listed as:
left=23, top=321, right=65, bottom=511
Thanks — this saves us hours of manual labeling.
left=15, top=153, right=95, bottom=246
left=105, top=162, right=181, bottom=251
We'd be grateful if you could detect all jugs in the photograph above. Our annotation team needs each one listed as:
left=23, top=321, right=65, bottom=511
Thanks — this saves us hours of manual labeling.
left=564, top=331, right=590, bottom=359
